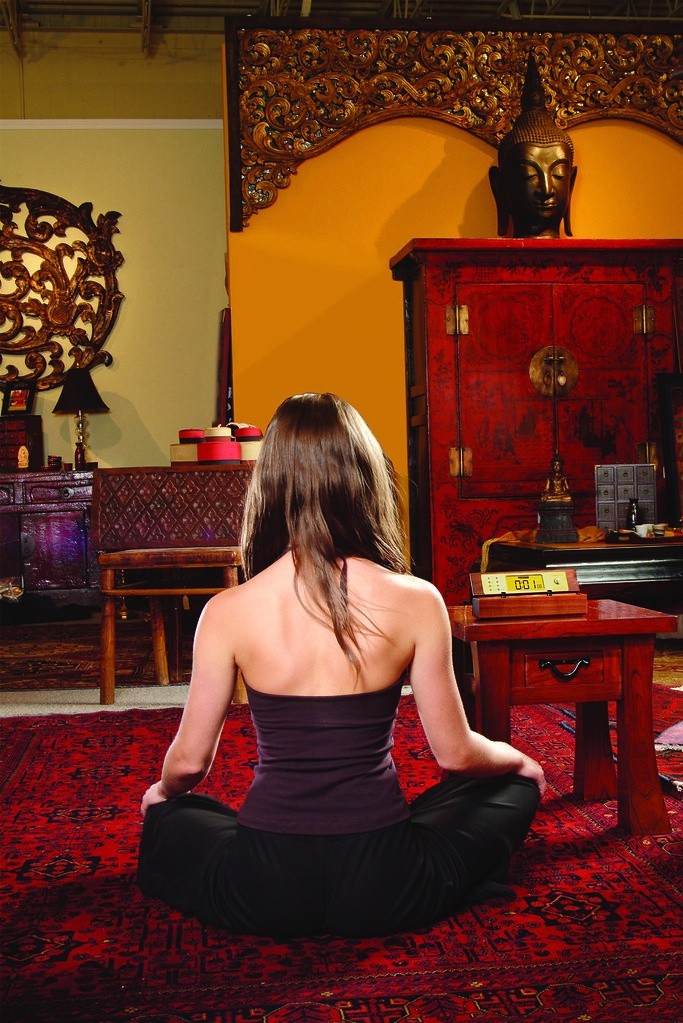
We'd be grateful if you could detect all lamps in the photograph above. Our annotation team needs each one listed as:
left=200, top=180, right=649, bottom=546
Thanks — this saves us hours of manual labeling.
left=52, top=368, right=110, bottom=471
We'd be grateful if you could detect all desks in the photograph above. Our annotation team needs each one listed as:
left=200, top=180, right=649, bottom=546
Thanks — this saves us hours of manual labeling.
left=97, top=546, right=242, bottom=706
left=472, top=538, right=683, bottom=651
left=447, top=599, right=679, bottom=836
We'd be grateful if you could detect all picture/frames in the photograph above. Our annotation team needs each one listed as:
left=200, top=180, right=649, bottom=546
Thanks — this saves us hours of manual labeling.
left=1, top=380, right=37, bottom=416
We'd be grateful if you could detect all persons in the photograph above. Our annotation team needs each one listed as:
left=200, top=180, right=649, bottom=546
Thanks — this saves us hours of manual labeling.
left=488, top=46, right=578, bottom=238
left=541, top=453, right=572, bottom=500
left=18, top=450, right=27, bottom=466
left=138, top=392, right=547, bottom=940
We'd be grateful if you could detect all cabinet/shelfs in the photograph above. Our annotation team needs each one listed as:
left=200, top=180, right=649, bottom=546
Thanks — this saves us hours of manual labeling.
left=0, top=470, right=120, bottom=608
left=389, top=237, right=683, bottom=606
left=0, top=414, right=45, bottom=470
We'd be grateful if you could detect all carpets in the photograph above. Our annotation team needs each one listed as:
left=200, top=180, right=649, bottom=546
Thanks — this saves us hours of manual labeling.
left=0, top=684, right=683, bottom=1023
